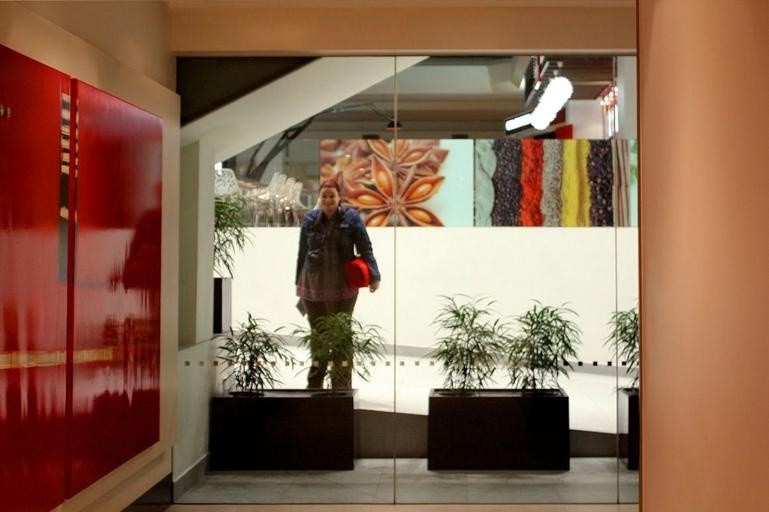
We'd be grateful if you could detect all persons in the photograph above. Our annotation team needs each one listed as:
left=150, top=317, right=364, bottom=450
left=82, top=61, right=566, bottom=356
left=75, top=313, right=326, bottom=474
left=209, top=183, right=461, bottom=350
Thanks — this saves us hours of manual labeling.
left=295, top=180, right=381, bottom=388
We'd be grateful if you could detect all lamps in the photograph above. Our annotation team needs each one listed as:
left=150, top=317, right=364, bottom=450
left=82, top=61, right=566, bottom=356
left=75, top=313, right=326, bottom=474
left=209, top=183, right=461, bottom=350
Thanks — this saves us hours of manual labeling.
left=328, top=102, right=405, bottom=131
left=530, top=74, right=574, bottom=131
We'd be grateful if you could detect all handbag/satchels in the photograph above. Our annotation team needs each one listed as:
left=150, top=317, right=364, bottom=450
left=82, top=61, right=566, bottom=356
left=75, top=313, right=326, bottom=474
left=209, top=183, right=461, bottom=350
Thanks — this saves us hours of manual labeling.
left=342, top=256, right=371, bottom=289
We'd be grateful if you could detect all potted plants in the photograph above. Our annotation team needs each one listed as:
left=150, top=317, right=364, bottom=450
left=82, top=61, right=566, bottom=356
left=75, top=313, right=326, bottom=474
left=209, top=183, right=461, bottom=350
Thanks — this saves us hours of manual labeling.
left=205, top=312, right=389, bottom=474
left=425, top=289, right=640, bottom=474
